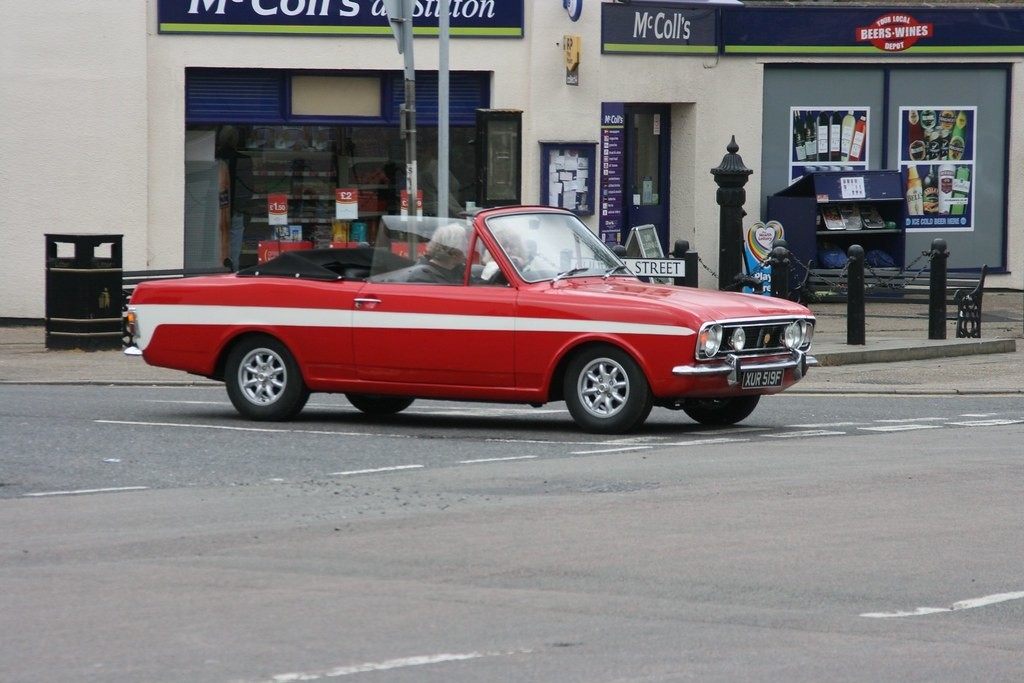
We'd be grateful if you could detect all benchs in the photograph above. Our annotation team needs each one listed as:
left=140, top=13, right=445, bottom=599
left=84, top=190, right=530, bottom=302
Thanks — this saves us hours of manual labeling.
left=788, top=262, right=991, bottom=339
left=361, top=264, right=482, bottom=284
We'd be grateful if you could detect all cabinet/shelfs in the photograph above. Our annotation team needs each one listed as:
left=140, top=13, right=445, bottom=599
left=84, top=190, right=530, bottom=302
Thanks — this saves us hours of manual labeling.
left=768, top=165, right=909, bottom=301
left=337, top=158, right=404, bottom=253
left=231, top=151, right=340, bottom=271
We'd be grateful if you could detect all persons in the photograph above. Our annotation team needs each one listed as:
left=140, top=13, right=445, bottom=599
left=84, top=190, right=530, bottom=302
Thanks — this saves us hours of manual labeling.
left=215, top=125, right=254, bottom=271
left=406, top=224, right=525, bottom=285
left=416, top=142, right=464, bottom=219
left=476, top=228, right=529, bottom=286
left=383, top=139, right=406, bottom=216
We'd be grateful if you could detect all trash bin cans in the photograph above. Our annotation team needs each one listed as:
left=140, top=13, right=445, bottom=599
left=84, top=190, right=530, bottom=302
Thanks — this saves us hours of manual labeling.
left=43, top=232, right=124, bottom=353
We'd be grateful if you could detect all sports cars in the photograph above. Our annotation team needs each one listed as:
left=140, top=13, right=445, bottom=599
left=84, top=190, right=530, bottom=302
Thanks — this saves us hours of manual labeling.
left=122, top=205, right=821, bottom=432
